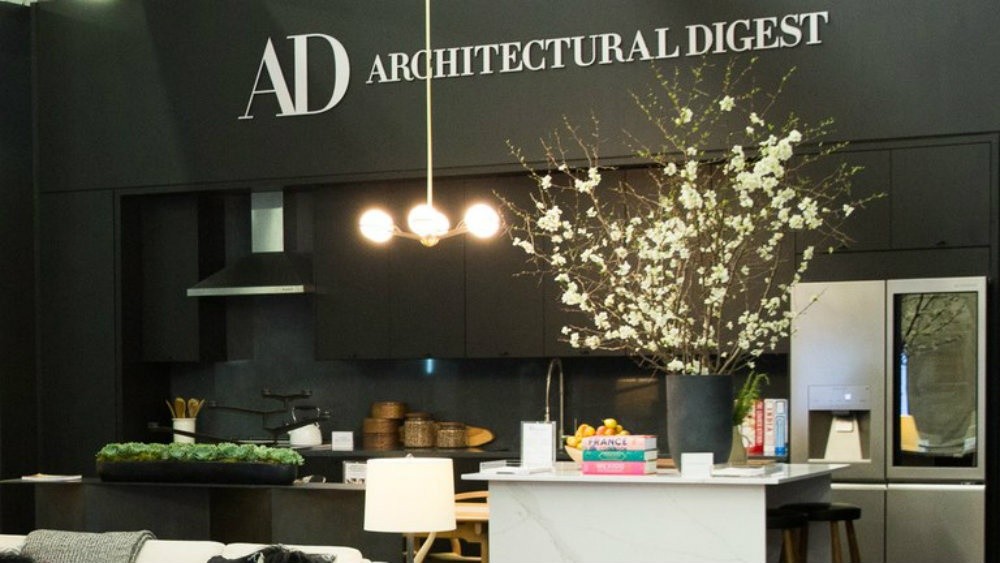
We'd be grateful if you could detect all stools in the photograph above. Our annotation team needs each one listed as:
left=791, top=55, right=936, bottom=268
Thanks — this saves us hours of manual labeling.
left=780, top=503, right=861, bottom=563
left=766, top=508, right=807, bottom=563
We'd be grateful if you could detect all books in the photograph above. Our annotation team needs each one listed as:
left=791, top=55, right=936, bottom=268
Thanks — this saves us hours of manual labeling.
left=22, top=472, right=83, bottom=481
left=582, top=435, right=658, bottom=475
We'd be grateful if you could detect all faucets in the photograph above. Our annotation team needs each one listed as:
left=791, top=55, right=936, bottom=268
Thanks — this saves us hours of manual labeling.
left=543, top=358, right=565, bottom=449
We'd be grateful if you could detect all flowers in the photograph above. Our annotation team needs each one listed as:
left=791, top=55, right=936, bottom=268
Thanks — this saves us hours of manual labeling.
left=487, top=44, right=887, bottom=375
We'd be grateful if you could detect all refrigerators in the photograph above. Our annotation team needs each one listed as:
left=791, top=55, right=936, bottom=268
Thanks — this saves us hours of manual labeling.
left=790, top=275, right=988, bottom=562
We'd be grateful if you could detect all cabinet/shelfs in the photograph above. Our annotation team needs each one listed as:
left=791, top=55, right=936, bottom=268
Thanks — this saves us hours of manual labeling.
left=315, top=132, right=1000, bottom=360
left=137, top=195, right=229, bottom=366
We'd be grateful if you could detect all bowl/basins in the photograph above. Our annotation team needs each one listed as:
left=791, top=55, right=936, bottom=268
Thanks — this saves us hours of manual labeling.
left=563, top=445, right=582, bottom=463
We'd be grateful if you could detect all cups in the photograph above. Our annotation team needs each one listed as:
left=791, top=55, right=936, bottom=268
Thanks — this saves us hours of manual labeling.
left=172, top=418, right=195, bottom=444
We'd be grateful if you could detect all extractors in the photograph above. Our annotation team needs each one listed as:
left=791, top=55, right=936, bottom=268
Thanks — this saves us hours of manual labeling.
left=185, top=192, right=335, bottom=296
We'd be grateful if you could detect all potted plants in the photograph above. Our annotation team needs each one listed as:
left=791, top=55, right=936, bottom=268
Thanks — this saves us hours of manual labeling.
left=98, top=442, right=303, bottom=485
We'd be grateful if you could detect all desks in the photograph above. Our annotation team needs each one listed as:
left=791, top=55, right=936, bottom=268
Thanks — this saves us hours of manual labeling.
left=460, top=461, right=850, bottom=563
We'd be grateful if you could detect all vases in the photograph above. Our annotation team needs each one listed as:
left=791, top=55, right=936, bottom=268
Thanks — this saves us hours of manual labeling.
left=665, top=374, right=733, bottom=467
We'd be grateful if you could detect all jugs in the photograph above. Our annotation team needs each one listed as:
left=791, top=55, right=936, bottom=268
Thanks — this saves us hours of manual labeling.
left=728, top=426, right=751, bottom=464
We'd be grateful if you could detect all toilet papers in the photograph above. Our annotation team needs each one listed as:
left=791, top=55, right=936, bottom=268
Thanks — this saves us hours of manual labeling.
left=519, top=420, right=557, bottom=472
left=680, top=451, right=714, bottom=480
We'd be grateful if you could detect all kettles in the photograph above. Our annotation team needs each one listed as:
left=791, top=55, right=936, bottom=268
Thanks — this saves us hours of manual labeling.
left=284, top=405, right=323, bottom=447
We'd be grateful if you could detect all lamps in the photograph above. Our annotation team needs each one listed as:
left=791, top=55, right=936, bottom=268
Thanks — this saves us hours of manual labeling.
left=356, top=0, right=499, bottom=244
left=363, top=454, right=456, bottom=563
